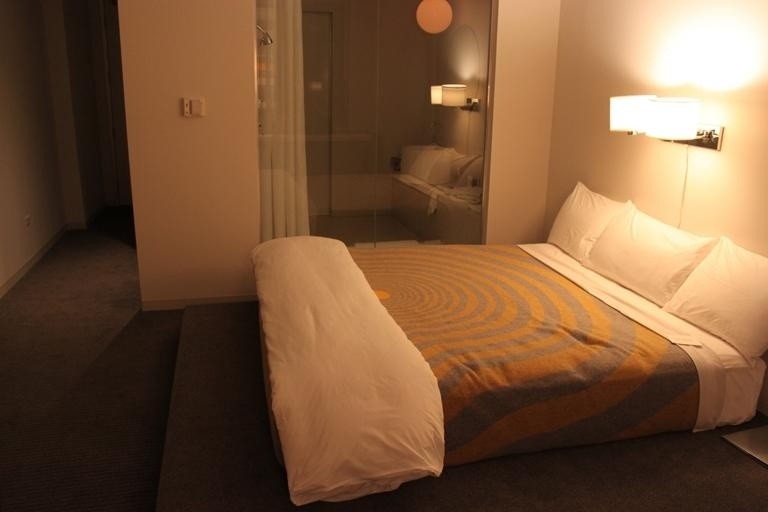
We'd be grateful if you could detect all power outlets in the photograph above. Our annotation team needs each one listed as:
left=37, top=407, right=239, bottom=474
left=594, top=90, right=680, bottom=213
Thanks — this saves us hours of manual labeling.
left=25, top=214, right=32, bottom=229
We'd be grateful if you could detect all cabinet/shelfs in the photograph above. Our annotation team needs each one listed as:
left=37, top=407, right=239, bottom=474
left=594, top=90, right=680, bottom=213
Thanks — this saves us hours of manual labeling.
left=117, top=0, right=260, bottom=311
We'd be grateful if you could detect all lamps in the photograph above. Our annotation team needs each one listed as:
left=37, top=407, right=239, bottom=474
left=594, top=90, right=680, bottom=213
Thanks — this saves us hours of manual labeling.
left=257, top=24, right=272, bottom=46
left=429, top=85, right=442, bottom=105
left=441, top=84, right=474, bottom=110
left=640, top=96, right=712, bottom=142
left=607, top=94, right=658, bottom=136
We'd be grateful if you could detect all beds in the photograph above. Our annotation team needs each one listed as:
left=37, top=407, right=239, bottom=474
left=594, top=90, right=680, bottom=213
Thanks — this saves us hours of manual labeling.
left=248, top=235, right=768, bottom=507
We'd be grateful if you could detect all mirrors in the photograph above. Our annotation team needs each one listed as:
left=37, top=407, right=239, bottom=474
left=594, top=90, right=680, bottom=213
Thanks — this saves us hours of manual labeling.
left=301, top=0, right=498, bottom=248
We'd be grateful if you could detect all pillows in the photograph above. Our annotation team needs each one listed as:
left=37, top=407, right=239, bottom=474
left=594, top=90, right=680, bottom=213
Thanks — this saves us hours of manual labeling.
left=424, top=147, right=480, bottom=190
left=579, top=199, right=718, bottom=309
left=408, top=141, right=453, bottom=180
left=544, top=179, right=623, bottom=266
left=449, top=156, right=483, bottom=200
left=659, top=234, right=767, bottom=365
